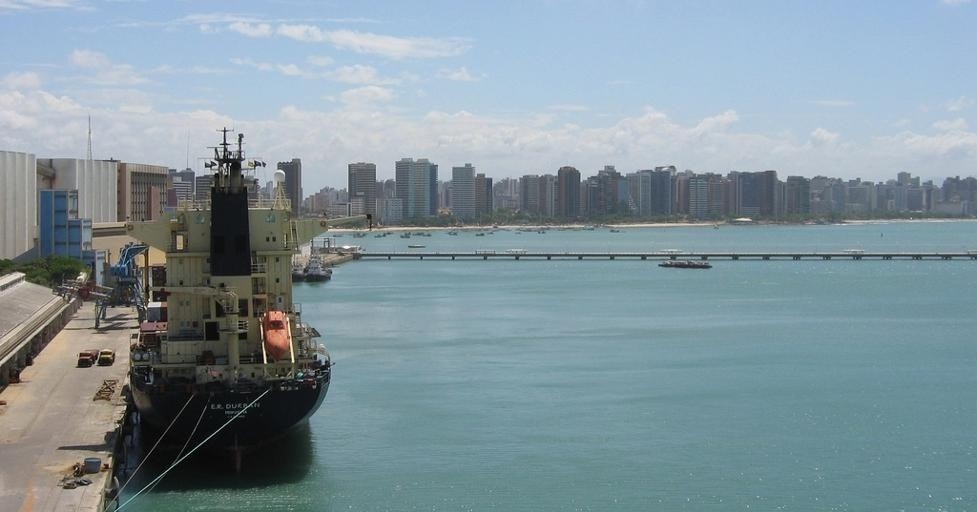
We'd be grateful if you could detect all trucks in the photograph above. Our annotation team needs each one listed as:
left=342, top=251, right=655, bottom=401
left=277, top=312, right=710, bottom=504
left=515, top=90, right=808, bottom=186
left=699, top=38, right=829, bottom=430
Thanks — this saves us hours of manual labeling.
left=77, top=348, right=99, bottom=367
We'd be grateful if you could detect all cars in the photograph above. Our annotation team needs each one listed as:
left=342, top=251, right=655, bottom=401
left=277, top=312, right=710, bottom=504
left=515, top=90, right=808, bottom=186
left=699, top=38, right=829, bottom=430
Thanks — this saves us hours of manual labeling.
left=100, top=349, right=115, bottom=365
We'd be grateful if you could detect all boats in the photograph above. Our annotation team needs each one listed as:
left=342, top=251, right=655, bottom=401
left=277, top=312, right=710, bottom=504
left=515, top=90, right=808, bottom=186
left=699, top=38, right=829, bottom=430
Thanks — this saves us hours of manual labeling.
left=352, top=224, right=620, bottom=248
left=303, top=257, right=333, bottom=280
left=124, top=125, right=372, bottom=431
left=658, top=260, right=714, bottom=268
